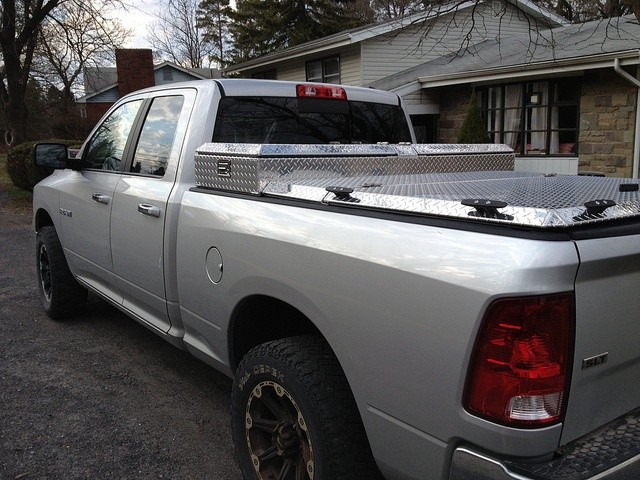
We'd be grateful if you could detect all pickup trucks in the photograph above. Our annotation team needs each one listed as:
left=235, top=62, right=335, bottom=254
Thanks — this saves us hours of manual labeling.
left=33, top=79, right=640, bottom=478
